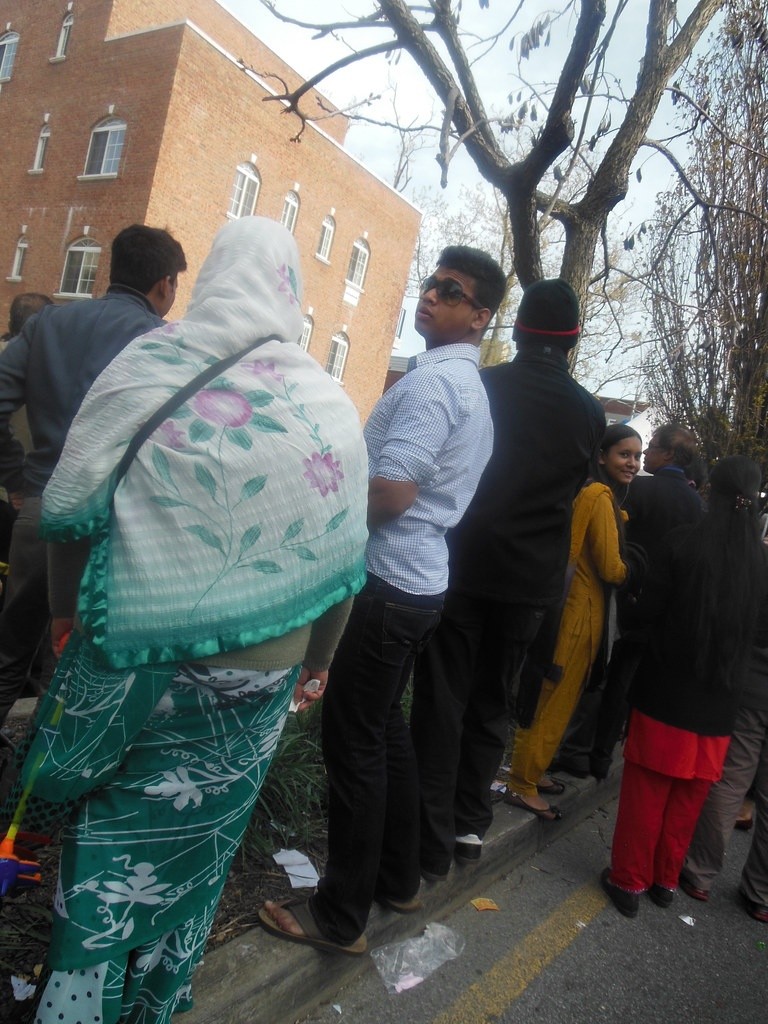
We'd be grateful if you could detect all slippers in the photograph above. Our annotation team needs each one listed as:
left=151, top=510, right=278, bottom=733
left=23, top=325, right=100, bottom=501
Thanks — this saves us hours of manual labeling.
left=386, top=898, right=419, bottom=911
left=260, top=899, right=368, bottom=956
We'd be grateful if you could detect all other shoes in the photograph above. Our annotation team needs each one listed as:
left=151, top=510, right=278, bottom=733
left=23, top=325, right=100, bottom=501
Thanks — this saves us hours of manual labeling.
left=649, top=885, right=673, bottom=904
left=733, top=818, right=750, bottom=828
left=422, top=850, right=447, bottom=882
left=539, top=780, right=572, bottom=794
left=506, top=789, right=565, bottom=824
left=455, top=845, right=484, bottom=865
left=682, top=879, right=708, bottom=900
left=603, top=867, right=639, bottom=918
left=738, top=893, right=766, bottom=921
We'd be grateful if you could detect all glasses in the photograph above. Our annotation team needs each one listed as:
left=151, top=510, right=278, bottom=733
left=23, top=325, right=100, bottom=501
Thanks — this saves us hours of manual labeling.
left=417, top=277, right=482, bottom=311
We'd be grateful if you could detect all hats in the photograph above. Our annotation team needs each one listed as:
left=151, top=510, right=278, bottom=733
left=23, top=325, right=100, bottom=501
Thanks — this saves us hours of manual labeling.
left=511, top=279, right=581, bottom=342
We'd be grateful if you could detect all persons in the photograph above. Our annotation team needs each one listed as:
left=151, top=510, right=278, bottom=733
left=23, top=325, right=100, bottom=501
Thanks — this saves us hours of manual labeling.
left=1, top=216, right=765, bottom=1022
